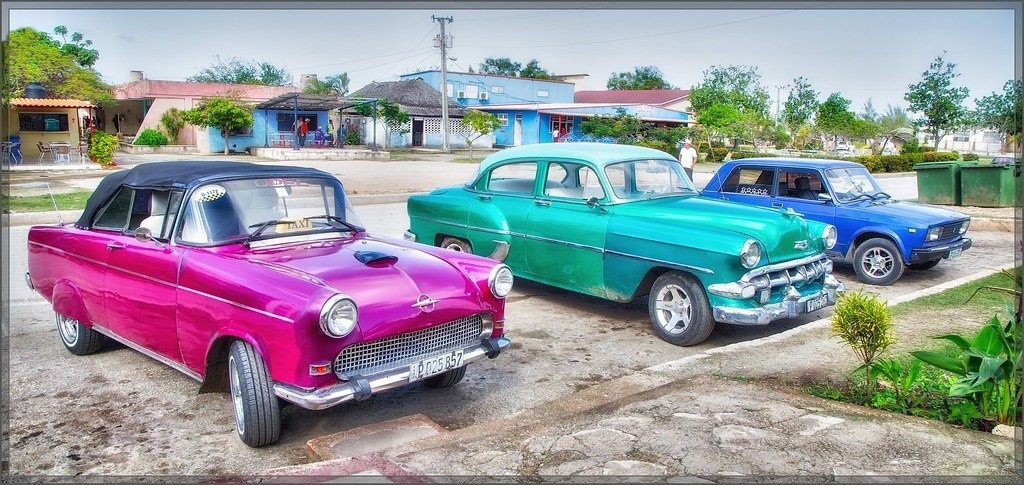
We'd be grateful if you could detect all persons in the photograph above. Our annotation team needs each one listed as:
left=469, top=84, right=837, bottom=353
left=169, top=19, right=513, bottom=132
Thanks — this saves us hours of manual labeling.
left=84, top=114, right=101, bottom=129
left=112, top=115, right=124, bottom=132
left=290, top=116, right=310, bottom=147
left=334, top=125, right=347, bottom=145
left=552, top=125, right=566, bottom=142
left=678, top=139, right=697, bottom=183
left=314, top=125, right=326, bottom=146
left=327, top=119, right=334, bottom=146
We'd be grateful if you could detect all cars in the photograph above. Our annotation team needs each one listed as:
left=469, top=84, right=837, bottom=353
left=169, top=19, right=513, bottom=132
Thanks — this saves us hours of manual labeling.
left=991, top=157, right=1018, bottom=165
left=407, top=143, right=843, bottom=350
left=23, top=158, right=511, bottom=446
left=689, top=161, right=975, bottom=289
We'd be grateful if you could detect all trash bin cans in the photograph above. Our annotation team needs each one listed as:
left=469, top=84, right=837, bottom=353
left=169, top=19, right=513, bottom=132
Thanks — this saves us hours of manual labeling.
left=912, top=160, right=979, bottom=206
left=958, top=161, right=1024, bottom=208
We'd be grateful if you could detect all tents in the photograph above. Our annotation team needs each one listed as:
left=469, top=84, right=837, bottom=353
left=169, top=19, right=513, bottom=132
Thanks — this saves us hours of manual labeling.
left=3, top=97, right=98, bottom=163
left=254, top=92, right=380, bottom=152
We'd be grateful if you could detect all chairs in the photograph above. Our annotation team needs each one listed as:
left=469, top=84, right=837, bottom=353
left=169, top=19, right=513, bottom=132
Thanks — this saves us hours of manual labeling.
left=3, top=142, right=71, bottom=164
left=112, top=186, right=286, bottom=236
left=793, top=177, right=819, bottom=200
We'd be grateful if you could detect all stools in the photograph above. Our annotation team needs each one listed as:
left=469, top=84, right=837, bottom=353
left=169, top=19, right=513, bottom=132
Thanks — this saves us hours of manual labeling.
left=271, top=133, right=335, bottom=148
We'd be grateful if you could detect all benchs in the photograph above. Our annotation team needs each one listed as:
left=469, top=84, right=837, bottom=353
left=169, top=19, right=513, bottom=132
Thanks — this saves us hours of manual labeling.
left=545, top=184, right=625, bottom=199
left=488, top=179, right=564, bottom=193
left=724, top=184, right=772, bottom=195
